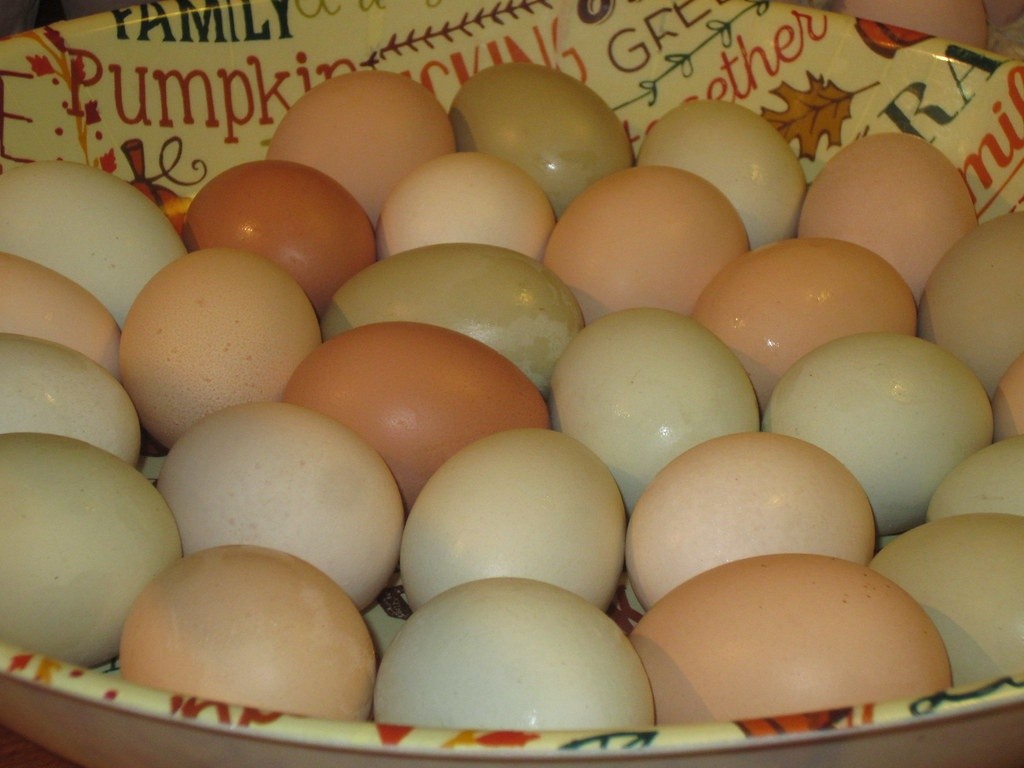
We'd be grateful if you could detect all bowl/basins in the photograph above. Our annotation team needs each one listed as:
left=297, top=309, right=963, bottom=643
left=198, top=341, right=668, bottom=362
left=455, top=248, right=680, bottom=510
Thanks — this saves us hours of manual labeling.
left=0, top=0, right=1024, bottom=768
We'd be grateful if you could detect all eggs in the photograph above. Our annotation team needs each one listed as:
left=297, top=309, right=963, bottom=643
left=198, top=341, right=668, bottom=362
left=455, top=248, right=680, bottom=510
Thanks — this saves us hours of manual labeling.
left=0, top=60, right=1024, bottom=732
